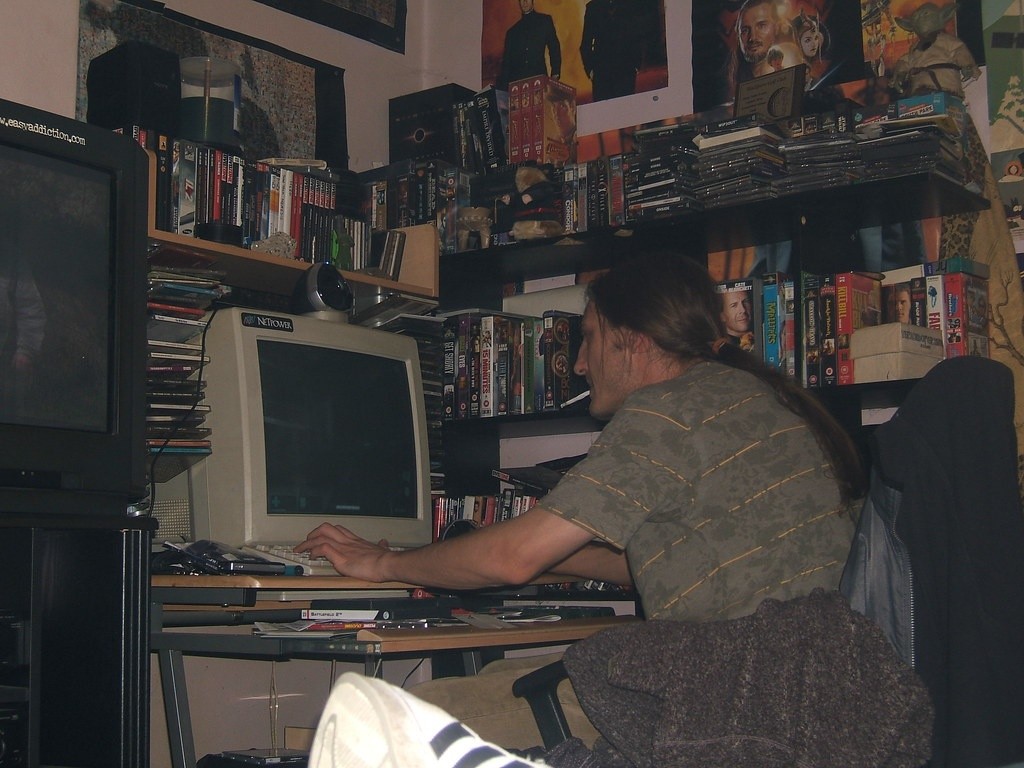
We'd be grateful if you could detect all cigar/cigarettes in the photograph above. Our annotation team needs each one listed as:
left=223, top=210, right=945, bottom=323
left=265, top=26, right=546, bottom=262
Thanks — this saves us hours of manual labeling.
left=560, top=390, right=590, bottom=408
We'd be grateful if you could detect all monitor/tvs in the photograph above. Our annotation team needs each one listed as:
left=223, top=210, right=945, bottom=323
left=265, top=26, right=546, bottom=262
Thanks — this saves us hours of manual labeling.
left=150, top=307, right=433, bottom=553
left=0, top=98, right=155, bottom=531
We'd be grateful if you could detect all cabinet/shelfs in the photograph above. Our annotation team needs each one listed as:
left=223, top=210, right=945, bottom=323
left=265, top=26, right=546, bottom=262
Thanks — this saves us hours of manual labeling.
left=140, top=149, right=991, bottom=549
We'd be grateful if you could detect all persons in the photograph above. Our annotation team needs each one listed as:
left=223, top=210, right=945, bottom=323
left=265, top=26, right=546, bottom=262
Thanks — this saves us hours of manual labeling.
left=839, top=335, right=848, bottom=348
left=971, top=340, right=981, bottom=356
left=824, top=339, right=834, bottom=355
left=546, top=87, right=577, bottom=165
left=470, top=337, right=479, bottom=352
left=502, top=0, right=561, bottom=87
left=889, top=3, right=981, bottom=98
left=482, top=331, right=492, bottom=345
left=294, top=261, right=860, bottom=752
left=717, top=285, right=754, bottom=349
left=736, top=0, right=821, bottom=89
left=579, top=0, right=651, bottom=101
left=948, top=319, right=961, bottom=343
left=968, top=290, right=987, bottom=326
left=886, top=285, right=913, bottom=324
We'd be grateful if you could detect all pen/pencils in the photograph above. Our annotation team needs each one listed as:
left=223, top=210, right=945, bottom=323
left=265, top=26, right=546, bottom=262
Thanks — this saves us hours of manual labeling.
left=161, top=543, right=220, bottom=568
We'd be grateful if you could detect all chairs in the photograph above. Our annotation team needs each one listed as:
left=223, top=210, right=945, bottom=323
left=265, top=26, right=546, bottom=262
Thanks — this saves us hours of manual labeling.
left=309, top=353, right=1023, bottom=768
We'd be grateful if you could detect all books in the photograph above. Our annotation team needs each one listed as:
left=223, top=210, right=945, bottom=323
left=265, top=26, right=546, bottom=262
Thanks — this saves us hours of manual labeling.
left=945, top=273, right=990, bottom=359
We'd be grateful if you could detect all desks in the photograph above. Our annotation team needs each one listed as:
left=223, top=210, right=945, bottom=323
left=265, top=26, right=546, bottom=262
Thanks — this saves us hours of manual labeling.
left=150, top=572, right=642, bottom=768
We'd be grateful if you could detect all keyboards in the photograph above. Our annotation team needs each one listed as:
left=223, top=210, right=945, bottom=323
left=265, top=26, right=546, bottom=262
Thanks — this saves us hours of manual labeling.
left=240, top=545, right=416, bottom=577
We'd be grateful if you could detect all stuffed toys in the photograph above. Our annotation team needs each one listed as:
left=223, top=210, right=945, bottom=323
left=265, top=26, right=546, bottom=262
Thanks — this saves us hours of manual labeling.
left=501, top=169, right=561, bottom=239
